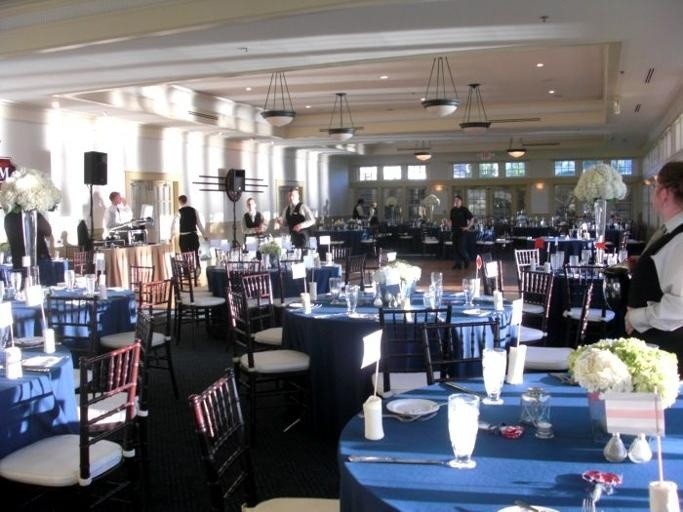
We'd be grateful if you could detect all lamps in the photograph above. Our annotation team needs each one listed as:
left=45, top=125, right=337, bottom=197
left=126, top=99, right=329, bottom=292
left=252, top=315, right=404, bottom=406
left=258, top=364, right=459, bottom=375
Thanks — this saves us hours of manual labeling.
left=260, top=72, right=295, bottom=127
left=412, top=140, right=432, bottom=161
left=459, top=83, right=490, bottom=135
left=421, top=56, right=461, bottom=116
left=328, top=93, right=357, bottom=142
left=507, top=137, right=527, bottom=159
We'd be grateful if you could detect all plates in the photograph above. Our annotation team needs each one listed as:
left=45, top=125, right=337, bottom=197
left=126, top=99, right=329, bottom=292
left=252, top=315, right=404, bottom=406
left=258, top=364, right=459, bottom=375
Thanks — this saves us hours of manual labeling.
left=14, top=336, right=44, bottom=349
left=288, top=302, right=314, bottom=308
left=21, top=355, right=63, bottom=372
left=386, top=398, right=440, bottom=416
left=347, top=313, right=375, bottom=320
left=462, top=308, right=491, bottom=317
left=498, top=504, right=557, bottom=512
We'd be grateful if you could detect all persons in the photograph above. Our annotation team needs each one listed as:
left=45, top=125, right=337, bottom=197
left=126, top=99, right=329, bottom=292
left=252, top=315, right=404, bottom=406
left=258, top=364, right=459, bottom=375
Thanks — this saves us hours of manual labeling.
left=450, top=195, right=474, bottom=269
left=368, top=200, right=379, bottom=225
left=4, top=205, right=58, bottom=287
left=240, top=198, right=267, bottom=248
left=171, top=195, right=208, bottom=277
left=103, top=192, right=133, bottom=245
left=278, top=186, right=315, bottom=248
left=613, top=160, right=682, bottom=376
left=353, top=198, right=366, bottom=217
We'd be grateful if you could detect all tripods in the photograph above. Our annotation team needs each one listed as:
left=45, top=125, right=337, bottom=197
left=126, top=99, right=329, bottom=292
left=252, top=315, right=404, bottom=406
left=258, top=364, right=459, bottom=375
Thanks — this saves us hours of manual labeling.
left=228, top=225, right=246, bottom=254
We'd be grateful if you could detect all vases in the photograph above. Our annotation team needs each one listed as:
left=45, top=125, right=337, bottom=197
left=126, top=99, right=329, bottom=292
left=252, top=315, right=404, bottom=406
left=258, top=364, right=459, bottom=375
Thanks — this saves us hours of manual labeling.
left=594, top=200, right=607, bottom=264
left=429, top=206, right=434, bottom=223
left=21, top=209, right=37, bottom=287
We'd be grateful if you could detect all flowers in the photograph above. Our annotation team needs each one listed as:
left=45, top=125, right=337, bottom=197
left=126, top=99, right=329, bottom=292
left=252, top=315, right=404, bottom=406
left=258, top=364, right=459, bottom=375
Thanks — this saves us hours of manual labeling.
left=423, top=194, right=440, bottom=208
left=573, top=164, right=627, bottom=205
left=0, top=166, right=62, bottom=215
left=385, top=197, right=397, bottom=209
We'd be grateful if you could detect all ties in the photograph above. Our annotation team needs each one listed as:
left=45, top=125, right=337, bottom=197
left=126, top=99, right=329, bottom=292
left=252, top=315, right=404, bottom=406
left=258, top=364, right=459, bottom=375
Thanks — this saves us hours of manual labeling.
left=117, top=208, right=122, bottom=225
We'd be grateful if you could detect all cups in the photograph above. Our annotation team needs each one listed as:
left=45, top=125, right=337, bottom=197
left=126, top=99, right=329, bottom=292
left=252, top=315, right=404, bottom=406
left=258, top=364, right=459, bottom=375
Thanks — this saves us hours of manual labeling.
left=482, top=347, right=507, bottom=406
left=345, top=285, right=358, bottom=315
left=11, top=272, right=22, bottom=296
left=422, top=271, right=481, bottom=311
left=329, top=278, right=342, bottom=305
left=85, top=274, right=95, bottom=298
left=64, top=270, right=75, bottom=292
left=447, top=393, right=480, bottom=470
left=543, top=250, right=628, bottom=273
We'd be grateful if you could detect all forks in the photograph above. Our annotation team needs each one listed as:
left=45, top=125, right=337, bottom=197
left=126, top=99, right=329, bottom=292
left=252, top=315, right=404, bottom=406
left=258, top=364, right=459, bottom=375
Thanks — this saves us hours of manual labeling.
left=358, top=411, right=420, bottom=423
left=581, top=497, right=596, bottom=512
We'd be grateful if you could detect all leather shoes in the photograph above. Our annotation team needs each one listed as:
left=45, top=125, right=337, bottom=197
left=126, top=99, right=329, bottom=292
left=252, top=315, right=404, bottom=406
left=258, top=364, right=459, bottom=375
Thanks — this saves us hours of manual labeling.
left=464, top=261, right=470, bottom=270
left=452, top=263, right=462, bottom=270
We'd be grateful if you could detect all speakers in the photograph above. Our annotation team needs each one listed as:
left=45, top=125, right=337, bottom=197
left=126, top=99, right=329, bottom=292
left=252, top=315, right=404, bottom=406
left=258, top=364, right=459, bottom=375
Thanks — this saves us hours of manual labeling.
left=84, top=151, right=108, bottom=185
left=225, top=168, right=246, bottom=192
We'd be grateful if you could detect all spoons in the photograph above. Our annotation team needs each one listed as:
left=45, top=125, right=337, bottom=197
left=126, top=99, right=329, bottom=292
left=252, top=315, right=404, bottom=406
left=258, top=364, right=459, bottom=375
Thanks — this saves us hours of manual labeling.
left=418, top=412, right=437, bottom=422
